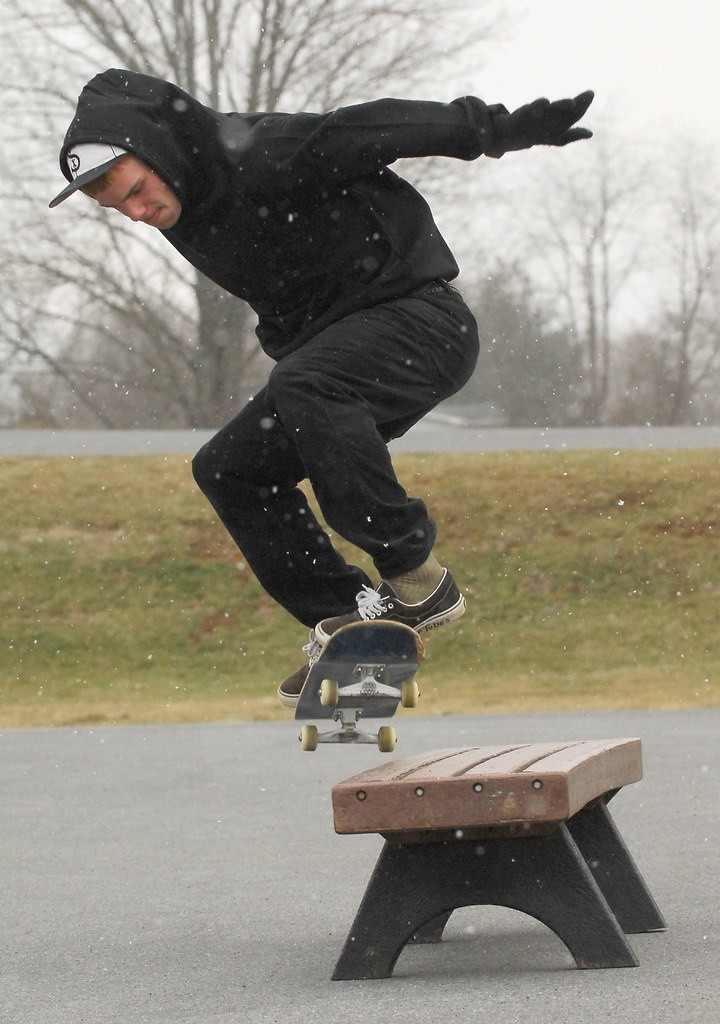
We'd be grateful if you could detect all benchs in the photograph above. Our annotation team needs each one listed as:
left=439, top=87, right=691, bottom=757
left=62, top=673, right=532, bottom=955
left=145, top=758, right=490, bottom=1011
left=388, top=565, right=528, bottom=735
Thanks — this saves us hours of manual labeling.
left=331, top=738, right=669, bottom=982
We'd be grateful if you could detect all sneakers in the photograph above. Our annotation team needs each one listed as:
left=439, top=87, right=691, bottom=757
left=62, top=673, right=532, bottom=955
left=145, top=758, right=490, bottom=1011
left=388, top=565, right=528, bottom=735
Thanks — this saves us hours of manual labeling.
left=314, top=566, right=467, bottom=649
left=277, top=628, right=325, bottom=708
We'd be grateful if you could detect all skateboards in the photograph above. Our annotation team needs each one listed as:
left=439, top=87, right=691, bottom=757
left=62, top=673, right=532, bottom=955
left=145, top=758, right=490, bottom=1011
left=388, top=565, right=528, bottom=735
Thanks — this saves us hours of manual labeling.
left=290, top=619, right=424, bottom=751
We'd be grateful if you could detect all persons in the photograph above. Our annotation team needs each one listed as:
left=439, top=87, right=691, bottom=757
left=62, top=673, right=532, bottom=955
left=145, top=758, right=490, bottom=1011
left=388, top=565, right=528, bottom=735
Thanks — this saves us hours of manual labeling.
left=48, top=68, right=595, bottom=707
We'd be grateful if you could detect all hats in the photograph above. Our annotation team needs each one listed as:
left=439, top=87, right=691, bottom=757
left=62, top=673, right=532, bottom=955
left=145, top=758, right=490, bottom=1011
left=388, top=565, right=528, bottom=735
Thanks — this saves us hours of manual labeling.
left=47, top=143, right=125, bottom=207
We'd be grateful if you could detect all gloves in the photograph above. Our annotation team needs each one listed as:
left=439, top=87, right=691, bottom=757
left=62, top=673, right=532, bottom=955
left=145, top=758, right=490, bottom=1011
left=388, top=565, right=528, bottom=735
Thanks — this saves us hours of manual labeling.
left=493, top=90, right=595, bottom=153
